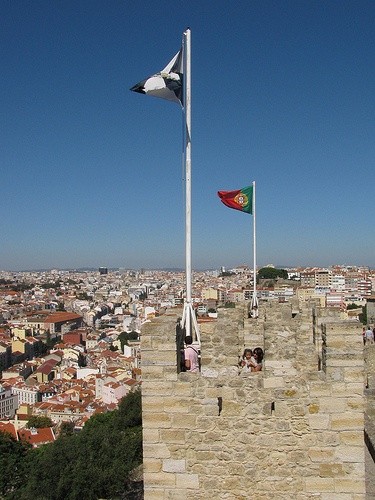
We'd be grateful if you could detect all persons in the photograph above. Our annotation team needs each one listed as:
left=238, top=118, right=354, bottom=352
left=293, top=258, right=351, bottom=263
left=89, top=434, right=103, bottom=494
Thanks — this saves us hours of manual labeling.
left=250, top=305, right=259, bottom=319
left=362, top=325, right=375, bottom=345
left=181, top=335, right=199, bottom=375
left=238, top=347, right=264, bottom=372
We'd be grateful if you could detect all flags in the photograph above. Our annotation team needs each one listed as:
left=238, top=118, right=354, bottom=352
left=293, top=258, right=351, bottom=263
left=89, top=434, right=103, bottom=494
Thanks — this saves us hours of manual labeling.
left=129, top=49, right=185, bottom=108
left=217, top=185, right=253, bottom=214
